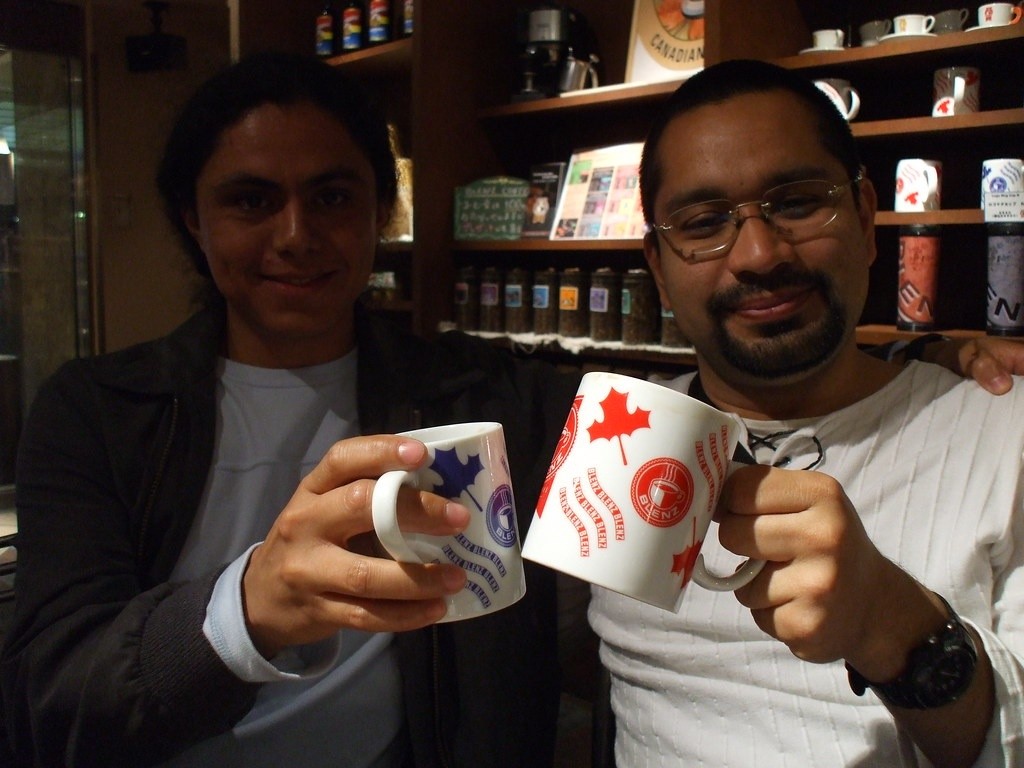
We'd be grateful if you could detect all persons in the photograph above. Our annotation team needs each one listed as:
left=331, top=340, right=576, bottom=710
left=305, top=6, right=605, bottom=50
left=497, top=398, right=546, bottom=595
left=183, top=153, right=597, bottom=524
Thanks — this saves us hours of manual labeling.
left=5, top=50, right=1024, bottom=768
left=583, top=55, right=1023, bottom=768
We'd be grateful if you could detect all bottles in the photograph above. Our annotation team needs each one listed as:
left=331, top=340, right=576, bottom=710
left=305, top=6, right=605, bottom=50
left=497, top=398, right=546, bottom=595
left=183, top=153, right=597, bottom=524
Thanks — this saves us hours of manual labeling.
left=311, top=1, right=699, bottom=348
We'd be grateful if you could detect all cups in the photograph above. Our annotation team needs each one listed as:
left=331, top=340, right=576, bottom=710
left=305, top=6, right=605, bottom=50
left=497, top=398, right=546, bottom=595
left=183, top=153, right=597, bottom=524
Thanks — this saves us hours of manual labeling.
left=352, top=419, right=528, bottom=626
left=795, top=3, right=1024, bottom=210
left=522, top=372, right=768, bottom=615
left=895, top=222, right=942, bottom=331
left=985, top=221, right=1024, bottom=337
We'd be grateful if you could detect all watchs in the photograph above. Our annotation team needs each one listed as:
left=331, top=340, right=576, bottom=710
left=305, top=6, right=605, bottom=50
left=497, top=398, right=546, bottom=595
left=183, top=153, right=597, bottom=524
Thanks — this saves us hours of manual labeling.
left=842, top=591, right=980, bottom=710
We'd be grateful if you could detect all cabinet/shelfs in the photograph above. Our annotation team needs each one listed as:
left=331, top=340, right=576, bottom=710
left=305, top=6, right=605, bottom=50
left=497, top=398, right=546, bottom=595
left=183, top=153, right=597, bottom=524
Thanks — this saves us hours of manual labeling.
left=228, top=0, right=1024, bottom=380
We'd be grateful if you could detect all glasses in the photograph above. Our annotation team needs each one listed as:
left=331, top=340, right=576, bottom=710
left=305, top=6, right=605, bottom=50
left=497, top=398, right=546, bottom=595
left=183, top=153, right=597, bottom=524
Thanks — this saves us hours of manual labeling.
left=647, top=170, right=863, bottom=255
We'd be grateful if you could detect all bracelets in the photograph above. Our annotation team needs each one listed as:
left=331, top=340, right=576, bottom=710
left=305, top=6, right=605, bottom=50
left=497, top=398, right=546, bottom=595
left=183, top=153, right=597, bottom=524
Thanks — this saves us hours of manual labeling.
left=904, top=332, right=953, bottom=366
